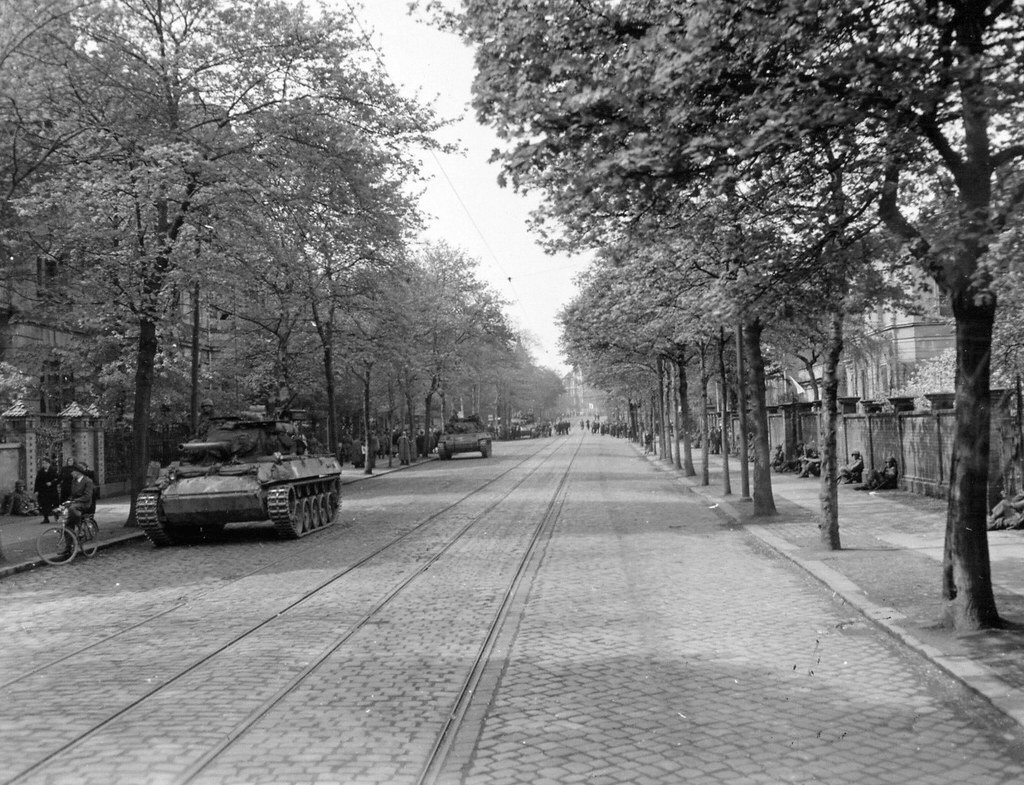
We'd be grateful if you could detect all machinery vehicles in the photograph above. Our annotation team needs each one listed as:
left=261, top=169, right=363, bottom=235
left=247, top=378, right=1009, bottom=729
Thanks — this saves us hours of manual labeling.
left=436, top=414, right=494, bottom=460
left=135, top=391, right=347, bottom=548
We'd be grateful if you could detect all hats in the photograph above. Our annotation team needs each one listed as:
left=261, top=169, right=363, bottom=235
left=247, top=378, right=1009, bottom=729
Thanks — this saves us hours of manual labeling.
left=69, top=464, right=85, bottom=474
left=15, top=479, right=26, bottom=488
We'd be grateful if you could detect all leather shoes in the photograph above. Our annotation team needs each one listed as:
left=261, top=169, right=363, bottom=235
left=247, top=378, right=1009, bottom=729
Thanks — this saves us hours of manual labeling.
left=694, top=445, right=876, bottom=492
left=40, top=519, right=50, bottom=524
left=57, top=546, right=71, bottom=555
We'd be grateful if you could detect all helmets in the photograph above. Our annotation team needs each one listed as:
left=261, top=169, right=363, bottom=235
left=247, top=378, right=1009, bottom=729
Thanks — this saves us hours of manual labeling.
left=775, top=440, right=896, bottom=463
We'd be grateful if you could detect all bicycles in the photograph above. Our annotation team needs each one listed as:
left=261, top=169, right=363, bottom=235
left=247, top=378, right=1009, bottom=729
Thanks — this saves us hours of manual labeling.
left=38, top=499, right=100, bottom=567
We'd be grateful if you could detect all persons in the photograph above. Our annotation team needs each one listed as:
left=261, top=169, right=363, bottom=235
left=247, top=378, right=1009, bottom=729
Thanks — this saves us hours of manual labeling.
left=337, top=410, right=823, bottom=480
left=56, top=464, right=94, bottom=555
left=54, top=456, right=81, bottom=507
left=836, top=450, right=864, bottom=485
left=3, top=479, right=41, bottom=517
left=852, top=456, right=898, bottom=491
left=33, top=456, right=59, bottom=524
left=193, top=398, right=223, bottom=443
left=78, top=461, right=100, bottom=532
left=985, top=486, right=1024, bottom=532
